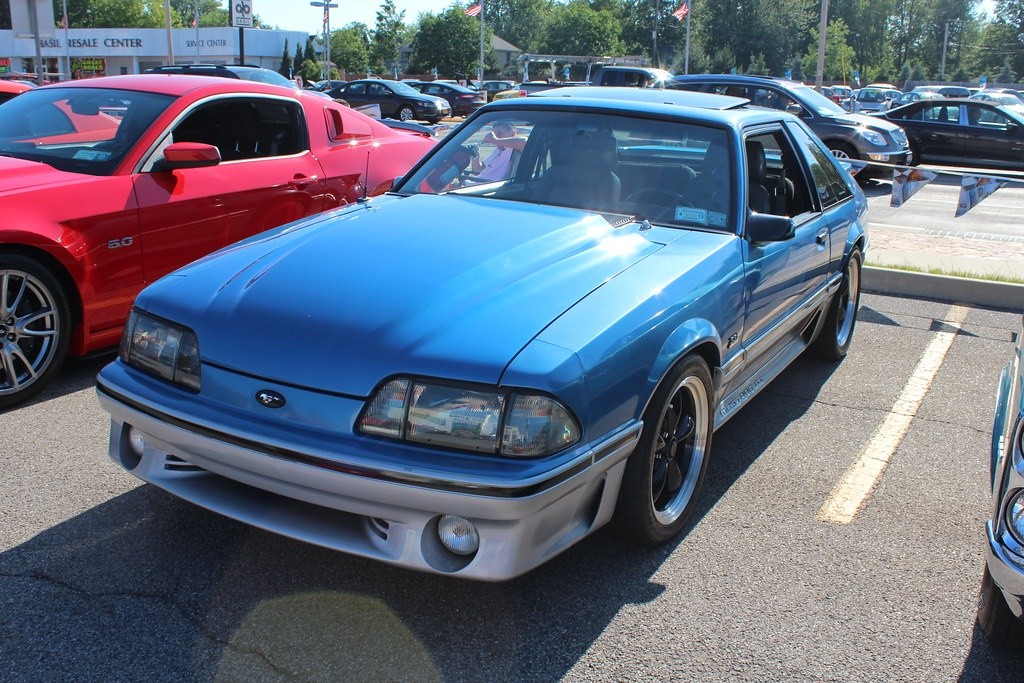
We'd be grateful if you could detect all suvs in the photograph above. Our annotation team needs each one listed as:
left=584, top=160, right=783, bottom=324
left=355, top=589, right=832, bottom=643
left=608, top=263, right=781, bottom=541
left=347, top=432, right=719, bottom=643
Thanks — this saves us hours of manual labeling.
left=652, top=75, right=913, bottom=188
left=144, top=64, right=333, bottom=100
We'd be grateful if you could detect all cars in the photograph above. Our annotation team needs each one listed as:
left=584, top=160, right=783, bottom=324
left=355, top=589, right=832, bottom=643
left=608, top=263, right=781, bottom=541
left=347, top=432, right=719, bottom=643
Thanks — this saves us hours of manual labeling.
left=493, top=83, right=522, bottom=101
left=809, top=84, right=904, bottom=113
left=910, top=85, right=1024, bottom=103
left=865, top=98, right=1024, bottom=172
left=967, top=93, right=1024, bottom=124
left=979, top=314, right=1024, bottom=649
left=890, top=92, right=959, bottom=118
left=400, top=79, right=516, bottom=118
left=0, top=75, right=451, bottom=412
left=324, top=79, right=452, bottom=124
left=304, top=80, right=356, bottom=92
left=1, top=80, right=121, bottom=147
left=96, top=86, right=868, bottom=583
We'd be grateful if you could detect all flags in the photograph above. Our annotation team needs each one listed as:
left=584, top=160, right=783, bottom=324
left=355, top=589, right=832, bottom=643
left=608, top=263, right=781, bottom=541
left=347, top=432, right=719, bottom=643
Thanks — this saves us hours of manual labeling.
left=464, top=1, right=481, bottom=16
left=672, top=0, right=688, bottom=22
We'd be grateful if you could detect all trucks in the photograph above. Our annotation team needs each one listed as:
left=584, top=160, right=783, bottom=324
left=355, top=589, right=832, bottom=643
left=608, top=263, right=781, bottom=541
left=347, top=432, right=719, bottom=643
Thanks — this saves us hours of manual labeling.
left=517, top=53, right=674, bottom=94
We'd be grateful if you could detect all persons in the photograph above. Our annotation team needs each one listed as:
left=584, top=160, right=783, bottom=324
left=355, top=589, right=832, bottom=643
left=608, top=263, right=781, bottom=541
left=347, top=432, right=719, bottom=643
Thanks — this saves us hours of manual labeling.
left=451, top=121, right=528, bottom=188
left=646, top=77, right=654, bottom=88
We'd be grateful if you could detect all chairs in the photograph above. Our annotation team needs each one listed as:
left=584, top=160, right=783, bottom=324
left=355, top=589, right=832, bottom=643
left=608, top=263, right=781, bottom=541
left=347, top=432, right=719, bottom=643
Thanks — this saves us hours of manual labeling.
left=700, top=138, right=771, bottom=214
left=534, top=129, right=621, bottom=211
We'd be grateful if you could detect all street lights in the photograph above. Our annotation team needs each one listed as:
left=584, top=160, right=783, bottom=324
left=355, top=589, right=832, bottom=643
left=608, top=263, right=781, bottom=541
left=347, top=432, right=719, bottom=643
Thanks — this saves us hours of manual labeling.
left=310, top=2, right=338, bottom=81
